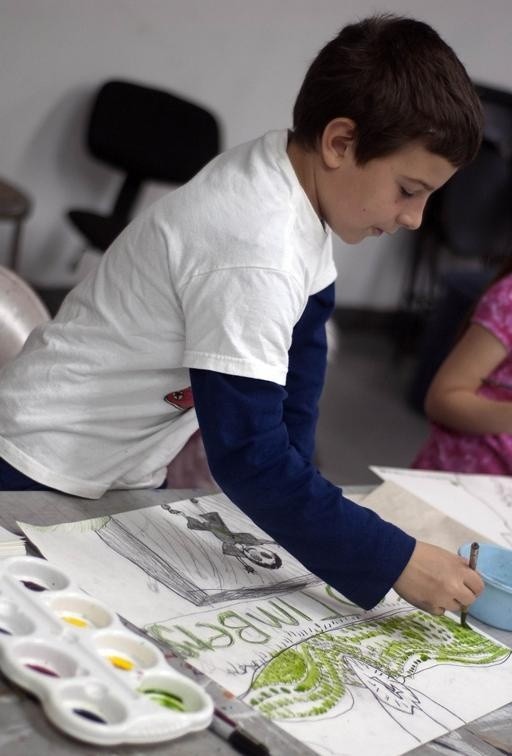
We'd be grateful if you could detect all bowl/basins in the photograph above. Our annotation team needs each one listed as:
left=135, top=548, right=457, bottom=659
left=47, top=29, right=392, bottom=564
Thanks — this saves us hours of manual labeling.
left=460, top=543, right=512, bottom=630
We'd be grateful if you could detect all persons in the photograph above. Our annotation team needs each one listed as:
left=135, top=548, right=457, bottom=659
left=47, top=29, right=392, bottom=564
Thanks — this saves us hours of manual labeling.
left=2, top=11, right=489, bottom=620
left=411, top=275, right=511, bottom=479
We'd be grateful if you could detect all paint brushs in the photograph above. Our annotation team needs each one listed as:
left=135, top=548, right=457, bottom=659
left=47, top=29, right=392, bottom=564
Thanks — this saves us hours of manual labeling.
left=460, top=543, right=479, bottom=630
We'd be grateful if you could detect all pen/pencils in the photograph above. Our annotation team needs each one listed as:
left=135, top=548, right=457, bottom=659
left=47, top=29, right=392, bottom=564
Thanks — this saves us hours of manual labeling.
left=209, top=706, right=272, bottom=756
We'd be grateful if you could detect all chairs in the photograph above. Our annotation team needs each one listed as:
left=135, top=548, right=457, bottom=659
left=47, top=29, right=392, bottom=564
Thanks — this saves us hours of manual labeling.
left=399, top=77, right=512, bottom=346
left=62, top=73, right=227, bottom=256
left=0, top=256, right=50, bottom=382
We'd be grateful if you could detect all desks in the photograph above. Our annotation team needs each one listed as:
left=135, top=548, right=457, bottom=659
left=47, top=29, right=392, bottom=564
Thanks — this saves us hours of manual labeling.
left=2, top=483, right=512, bottom=756
